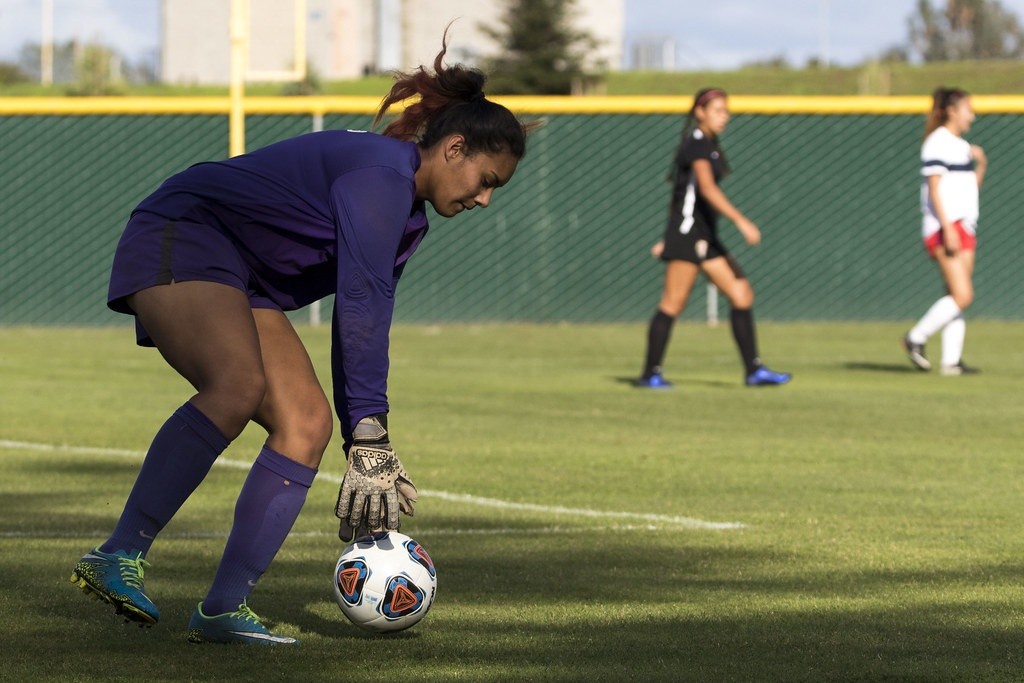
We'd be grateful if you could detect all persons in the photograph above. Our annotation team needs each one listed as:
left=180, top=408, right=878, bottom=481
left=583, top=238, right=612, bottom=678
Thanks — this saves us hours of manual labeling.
left=70, top=19, right=542, bottom=653
left=637, top=88, right=791, bottom=388
left=905, top=86, right=989, bottom=377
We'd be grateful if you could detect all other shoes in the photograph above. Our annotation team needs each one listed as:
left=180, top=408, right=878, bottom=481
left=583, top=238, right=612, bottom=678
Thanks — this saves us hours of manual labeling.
left=901, top=329, right=931, bottom=372
left=940, top=362, right=983, bottom=376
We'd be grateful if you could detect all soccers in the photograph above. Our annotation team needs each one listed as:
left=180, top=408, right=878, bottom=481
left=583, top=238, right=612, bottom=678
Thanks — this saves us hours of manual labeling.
left=333, top=530, right=437, bottom=634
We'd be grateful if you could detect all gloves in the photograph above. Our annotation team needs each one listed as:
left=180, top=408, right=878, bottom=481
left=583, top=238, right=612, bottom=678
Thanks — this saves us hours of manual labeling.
left=335, top=410, right=419, bottom=545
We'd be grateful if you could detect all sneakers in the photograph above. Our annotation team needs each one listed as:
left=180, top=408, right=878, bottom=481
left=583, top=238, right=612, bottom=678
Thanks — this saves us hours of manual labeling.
left=745, top=364, right=793, bottom=387
left=636, top=375, right=673, bottom=388
left=70, top=544, right=162, bottom=629
left=185, top=602, right=300, bottom=648
